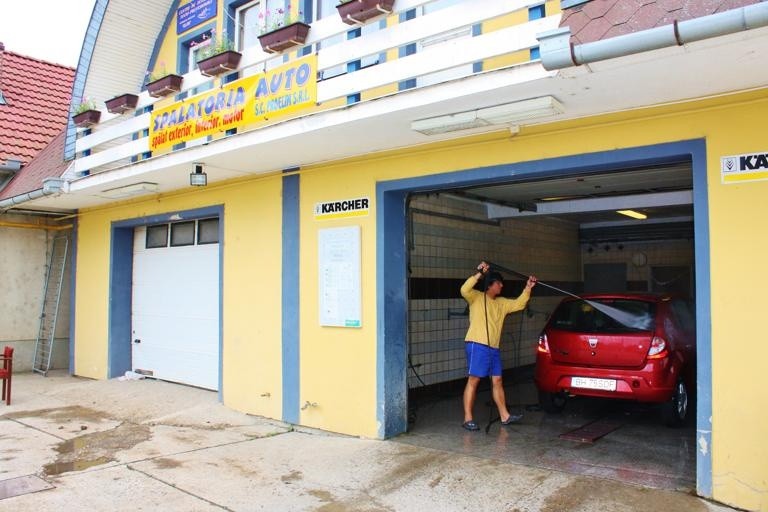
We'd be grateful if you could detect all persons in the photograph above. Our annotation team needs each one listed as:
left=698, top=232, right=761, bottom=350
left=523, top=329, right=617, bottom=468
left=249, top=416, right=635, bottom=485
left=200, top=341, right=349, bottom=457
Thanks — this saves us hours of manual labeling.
left=459, top=260, right=538, bottom=432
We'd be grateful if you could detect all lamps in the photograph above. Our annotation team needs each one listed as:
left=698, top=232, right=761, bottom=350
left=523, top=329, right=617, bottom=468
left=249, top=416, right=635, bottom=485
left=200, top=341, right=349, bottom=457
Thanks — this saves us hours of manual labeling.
left=411, top=95, right=565, bottom=136
left=190, top=163, right=207, bottom=186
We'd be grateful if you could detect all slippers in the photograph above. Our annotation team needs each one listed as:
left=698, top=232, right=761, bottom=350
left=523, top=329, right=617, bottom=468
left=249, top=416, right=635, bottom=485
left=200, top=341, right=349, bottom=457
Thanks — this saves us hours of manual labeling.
left=501, top=415, right=523, bottom=424
left=463, top=420, right=480, bottom=430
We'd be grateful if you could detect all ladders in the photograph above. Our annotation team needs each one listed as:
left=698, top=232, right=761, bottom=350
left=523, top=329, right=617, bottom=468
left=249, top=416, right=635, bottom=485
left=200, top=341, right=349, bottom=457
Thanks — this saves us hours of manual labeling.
left=32, top=234, right=70, bottom=375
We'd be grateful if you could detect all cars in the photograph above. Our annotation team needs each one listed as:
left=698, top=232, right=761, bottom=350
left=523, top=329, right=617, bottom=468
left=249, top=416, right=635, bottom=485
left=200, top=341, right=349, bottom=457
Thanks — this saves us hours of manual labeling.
left=535, top=292, right=697, bottom=428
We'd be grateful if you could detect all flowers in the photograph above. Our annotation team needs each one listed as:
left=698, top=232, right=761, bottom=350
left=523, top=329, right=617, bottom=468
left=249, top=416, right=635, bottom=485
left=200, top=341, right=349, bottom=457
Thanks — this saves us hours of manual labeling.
left=190, top=28, right=235, bottom=62
left=255, top=3, right=309, bottom=36
left=144, top=60, right=175, bottom=83
left=68, top=95, right=96, bottom=116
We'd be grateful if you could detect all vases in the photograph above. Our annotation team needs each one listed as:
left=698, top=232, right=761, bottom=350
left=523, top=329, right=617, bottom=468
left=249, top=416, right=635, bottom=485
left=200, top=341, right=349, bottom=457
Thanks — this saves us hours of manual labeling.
left=336, top=0, right=395, bottom=25
left=104, top=94, right=139, bottom=114
left=257, top=21, right=311, bottom=53
left=196, top=50, right=242, bottom=77
left=72, top=110, right=101, bottom=127
left=145, top=74, right=184, bottom=98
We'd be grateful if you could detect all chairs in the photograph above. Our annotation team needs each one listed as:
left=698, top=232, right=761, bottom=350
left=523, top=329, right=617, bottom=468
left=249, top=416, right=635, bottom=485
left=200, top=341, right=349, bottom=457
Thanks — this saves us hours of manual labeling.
left=0, top=345, right=13, bottom=405
